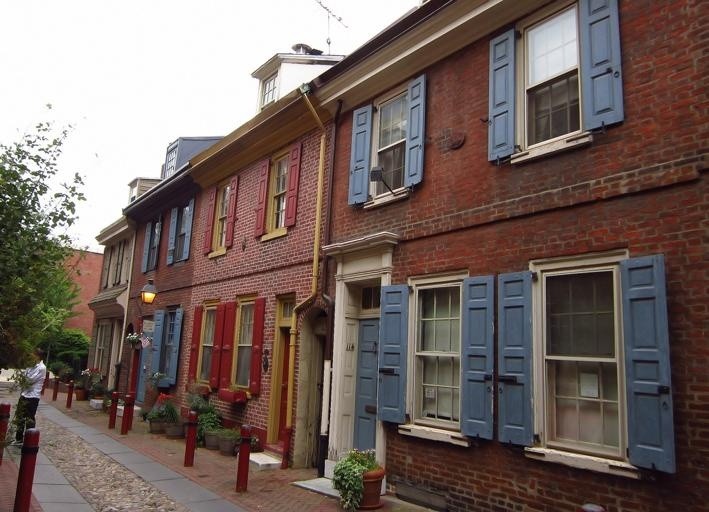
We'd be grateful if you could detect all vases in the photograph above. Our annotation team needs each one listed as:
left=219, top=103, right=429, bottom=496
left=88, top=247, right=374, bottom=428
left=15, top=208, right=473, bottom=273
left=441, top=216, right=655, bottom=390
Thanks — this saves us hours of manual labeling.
left=75, top=388, right=106, bottom=400
left=133, top=341, right=143, bottom=350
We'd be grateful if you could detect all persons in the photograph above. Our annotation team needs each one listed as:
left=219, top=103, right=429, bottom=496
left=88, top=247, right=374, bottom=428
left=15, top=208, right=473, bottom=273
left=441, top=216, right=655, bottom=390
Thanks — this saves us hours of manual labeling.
left=7, top=348, right=46, bottom=446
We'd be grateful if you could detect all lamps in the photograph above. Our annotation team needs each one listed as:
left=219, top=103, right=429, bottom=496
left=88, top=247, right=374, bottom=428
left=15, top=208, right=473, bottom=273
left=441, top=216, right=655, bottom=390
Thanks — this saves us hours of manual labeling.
left=136, top=278, right=158, bottom=305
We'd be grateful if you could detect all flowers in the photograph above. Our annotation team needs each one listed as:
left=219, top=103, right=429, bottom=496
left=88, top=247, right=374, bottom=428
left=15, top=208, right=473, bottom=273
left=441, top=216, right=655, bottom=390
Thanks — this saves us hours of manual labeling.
left=126, top=331, right=153, bottom=344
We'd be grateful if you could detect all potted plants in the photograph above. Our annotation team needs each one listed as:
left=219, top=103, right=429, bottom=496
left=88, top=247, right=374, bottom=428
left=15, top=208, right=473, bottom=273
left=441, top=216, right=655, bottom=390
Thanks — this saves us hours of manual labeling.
left=146, top=394, right=259, bottom=456
left=331, top=448, right=385, bottom=511
